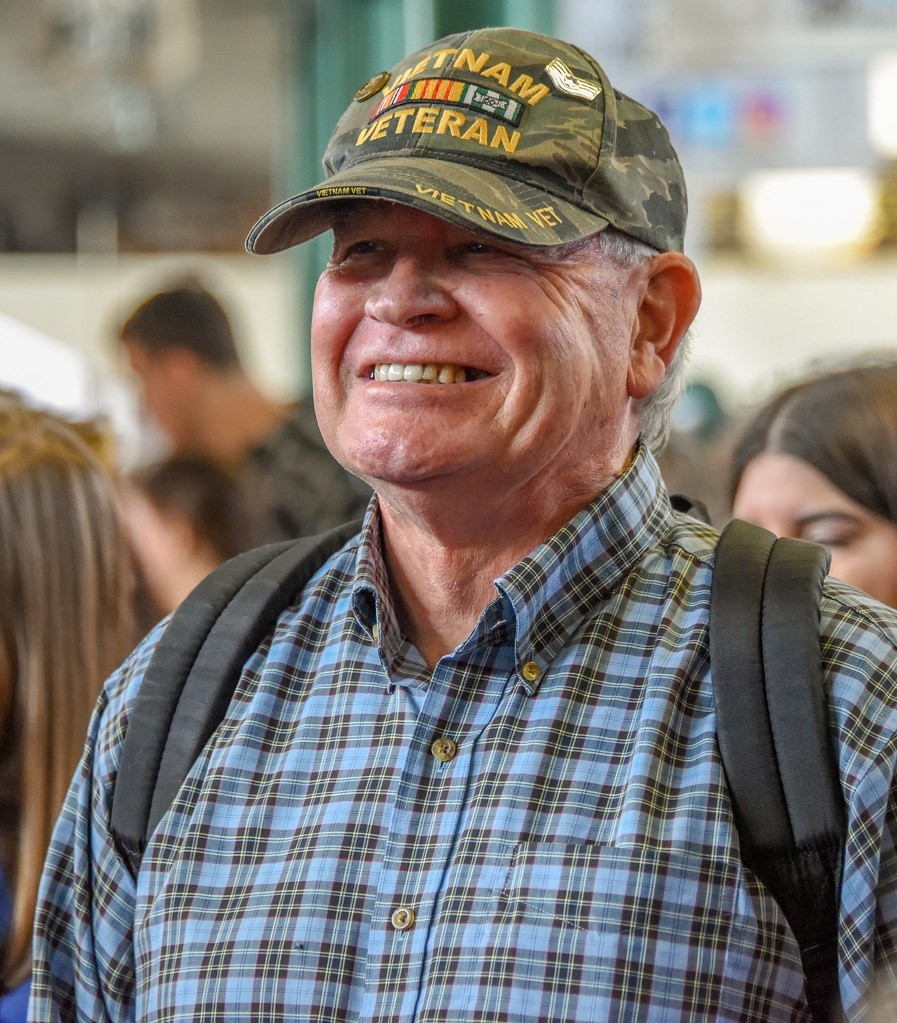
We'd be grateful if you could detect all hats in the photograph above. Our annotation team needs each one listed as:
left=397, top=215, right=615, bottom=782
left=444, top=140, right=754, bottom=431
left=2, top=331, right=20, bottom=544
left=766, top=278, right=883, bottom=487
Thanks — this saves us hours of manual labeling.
left=244, top=27, right=689, bottom=255
left=0, top=312, right=100, bottom=423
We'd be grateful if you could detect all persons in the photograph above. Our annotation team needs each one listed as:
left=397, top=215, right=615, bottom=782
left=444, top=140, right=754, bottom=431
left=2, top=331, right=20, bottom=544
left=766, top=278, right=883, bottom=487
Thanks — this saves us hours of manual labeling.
left=0, top=29, right=897, bottom=1023
